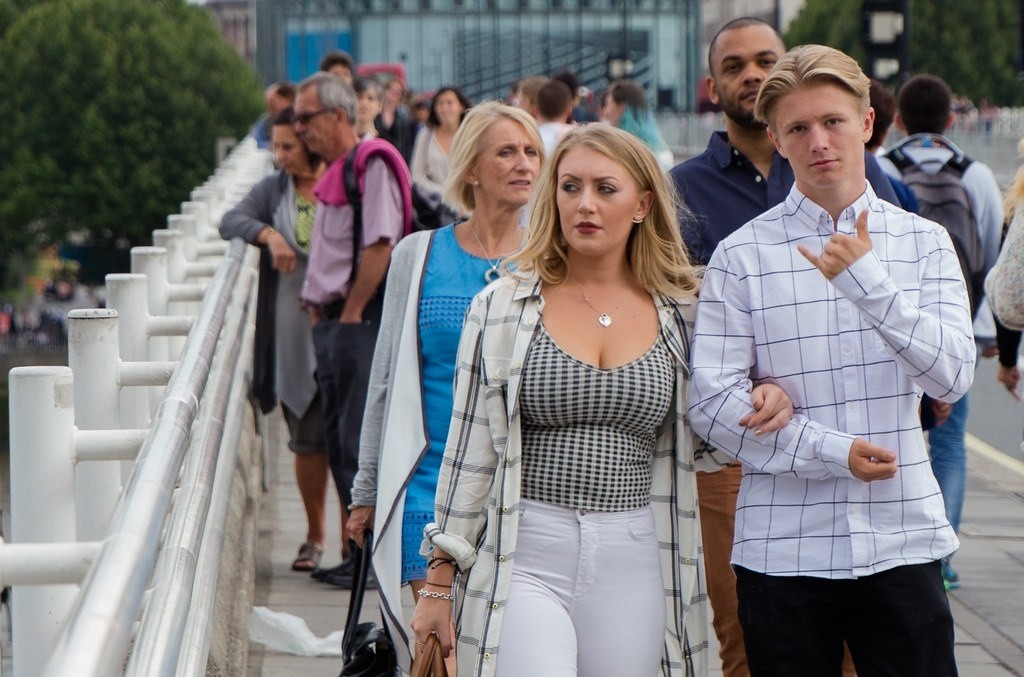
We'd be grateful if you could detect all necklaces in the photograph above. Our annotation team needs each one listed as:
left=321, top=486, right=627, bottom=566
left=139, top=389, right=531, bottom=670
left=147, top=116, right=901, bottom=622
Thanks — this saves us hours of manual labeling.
left=575, top=276, right=635, bottom=327
left=468, top=220, right=523, bottom=285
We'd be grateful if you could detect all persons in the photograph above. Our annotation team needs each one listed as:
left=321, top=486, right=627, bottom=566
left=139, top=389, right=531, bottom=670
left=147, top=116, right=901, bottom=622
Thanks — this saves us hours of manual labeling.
left=867, top=77, right=954, bottom=433
left=285, top=71, right=412, bottom=592
left=877, top=77, right=1024, bottom=590
left=344, top=101, right=546, bottom=677
left=252, top=51, right=674, bottom=179
left=412, top=124, right=797, bottom=677
left=219, top=105, right=331, bottom=571
left=656, top=15, right=903, bottom=276
left=688, top=44, right=980, bottom=677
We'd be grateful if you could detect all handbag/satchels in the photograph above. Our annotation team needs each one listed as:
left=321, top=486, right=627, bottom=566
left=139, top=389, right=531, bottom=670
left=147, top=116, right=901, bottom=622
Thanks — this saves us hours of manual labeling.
left=340, top=532, right=400, bottom=677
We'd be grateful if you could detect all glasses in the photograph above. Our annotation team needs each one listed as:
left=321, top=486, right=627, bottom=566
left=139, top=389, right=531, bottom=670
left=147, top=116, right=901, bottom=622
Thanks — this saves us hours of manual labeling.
left=294, top=108, right=330, bottom=127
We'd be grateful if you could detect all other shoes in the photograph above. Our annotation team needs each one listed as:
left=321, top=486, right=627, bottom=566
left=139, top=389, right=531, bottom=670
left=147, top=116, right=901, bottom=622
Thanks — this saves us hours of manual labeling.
left=941, top=563, right=961, bottom=590
left=310, top=546, right=379, bottom=592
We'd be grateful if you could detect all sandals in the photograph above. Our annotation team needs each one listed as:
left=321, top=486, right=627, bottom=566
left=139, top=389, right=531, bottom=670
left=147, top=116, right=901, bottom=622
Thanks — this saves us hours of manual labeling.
left=291, top=541, right=324, bottom=573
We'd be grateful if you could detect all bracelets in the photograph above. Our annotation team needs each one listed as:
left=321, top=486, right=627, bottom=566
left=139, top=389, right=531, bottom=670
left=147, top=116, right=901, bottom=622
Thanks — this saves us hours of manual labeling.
left=424, top=580, right=451, bottom=589
left=346, top=504, right=361, bottom=510
left=265, top=228, right=276, bottom=243
left=426, top=555, right=460, bottom=572
left=416, top=587, right=453, bottom=599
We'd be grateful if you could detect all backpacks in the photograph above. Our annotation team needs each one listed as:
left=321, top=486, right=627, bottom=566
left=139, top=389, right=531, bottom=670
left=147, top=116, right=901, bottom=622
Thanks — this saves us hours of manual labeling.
left=889, top=149, right=987, bottom=304
left=340, top=136, right=462, bottom=328
